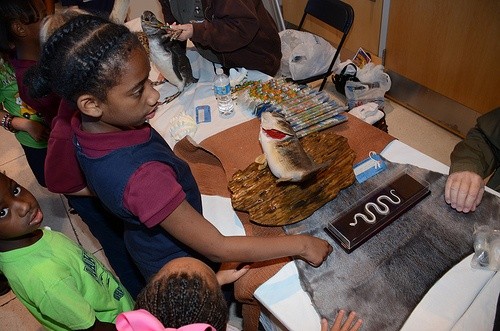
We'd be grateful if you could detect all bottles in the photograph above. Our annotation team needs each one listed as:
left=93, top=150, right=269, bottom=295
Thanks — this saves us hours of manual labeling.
left=213, top=68, right=236, bottom=119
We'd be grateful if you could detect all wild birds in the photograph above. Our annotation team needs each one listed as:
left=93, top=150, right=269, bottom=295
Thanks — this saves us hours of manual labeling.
left=139, top=10, right=200, bottom=105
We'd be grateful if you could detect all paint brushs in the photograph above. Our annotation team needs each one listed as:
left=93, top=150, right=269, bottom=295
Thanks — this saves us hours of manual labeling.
left=144, top=19, right=182, bottom=43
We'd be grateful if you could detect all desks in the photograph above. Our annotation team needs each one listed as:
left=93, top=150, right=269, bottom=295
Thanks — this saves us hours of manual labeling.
left=139, top=26, right=500, bottom=331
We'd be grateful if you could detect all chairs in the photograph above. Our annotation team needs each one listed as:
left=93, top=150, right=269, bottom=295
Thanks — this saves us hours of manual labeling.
left=282, top=0, right=356, bottom=91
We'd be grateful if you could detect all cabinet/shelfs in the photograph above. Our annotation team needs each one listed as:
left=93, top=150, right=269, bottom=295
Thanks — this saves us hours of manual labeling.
left=279, top=0, right=500, bottom=144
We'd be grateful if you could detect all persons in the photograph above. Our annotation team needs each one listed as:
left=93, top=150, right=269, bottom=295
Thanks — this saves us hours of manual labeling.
left=0, top=60, right=52, bottom=191
left=0, top=0, right=61, bottom=121
left=158, top=0, right=282, bottom=77
left=114, top=255, right=362, bottom=331
left=38, top=8, right=146, bottom=301
left=443, top=106, right=500, bottom=213
left=22, top=17, right=333, bottom=285
left=0, top=172, right=136, bottom=331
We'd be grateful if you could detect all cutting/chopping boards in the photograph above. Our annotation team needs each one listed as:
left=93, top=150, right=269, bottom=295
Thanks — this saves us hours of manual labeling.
left=226, top=132, right=356, bottom=227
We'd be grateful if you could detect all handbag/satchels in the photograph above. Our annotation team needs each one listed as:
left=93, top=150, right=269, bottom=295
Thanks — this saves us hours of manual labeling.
left=279, top=29, right=341, bottom=80
left=332, top=59, right=391, bottom=109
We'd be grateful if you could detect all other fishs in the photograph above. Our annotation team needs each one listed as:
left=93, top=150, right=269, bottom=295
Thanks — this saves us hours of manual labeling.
left=253, top=110, right=331, bottom=185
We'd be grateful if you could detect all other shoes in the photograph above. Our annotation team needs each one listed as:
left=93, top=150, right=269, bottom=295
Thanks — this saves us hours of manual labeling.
left=0, top=274, right=10, bottom=296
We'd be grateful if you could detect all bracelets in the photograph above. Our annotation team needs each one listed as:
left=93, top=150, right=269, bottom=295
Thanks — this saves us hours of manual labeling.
left=0, top=113, right=21, bottom=134
